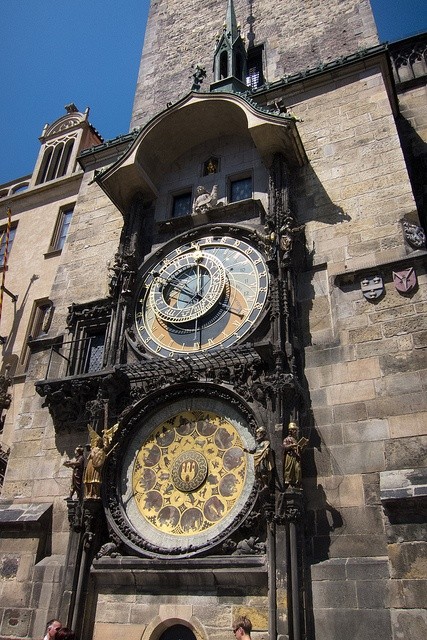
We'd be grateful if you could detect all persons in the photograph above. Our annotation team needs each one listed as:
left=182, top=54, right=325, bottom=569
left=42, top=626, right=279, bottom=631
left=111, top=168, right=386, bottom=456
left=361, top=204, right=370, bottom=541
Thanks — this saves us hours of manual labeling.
left=238, top=426, right=275, bottom=494
left=194, top=184, right=218, bottom=212
left=283, top=422, right=304, bottom=490
left=64, top=445, right=84, bottom=501
left=232, top=616, right=252, bottom=640
left=82, top=422, right=120, bottom=500
left=279, top=217, right=305, bottom=260
left=54, top=628, right=76, bottom=639
left=43, top=619, right=62, bottom=640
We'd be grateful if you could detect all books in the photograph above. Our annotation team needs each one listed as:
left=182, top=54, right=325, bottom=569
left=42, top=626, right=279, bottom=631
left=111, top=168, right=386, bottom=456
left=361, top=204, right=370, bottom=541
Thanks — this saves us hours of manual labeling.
left=296, top=437, right=309, bottom=447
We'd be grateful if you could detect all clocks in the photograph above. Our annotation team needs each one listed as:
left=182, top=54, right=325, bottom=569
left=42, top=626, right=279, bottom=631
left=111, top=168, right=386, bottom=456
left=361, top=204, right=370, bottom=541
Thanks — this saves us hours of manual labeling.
left=122, top=223, right=274, bottom=363
